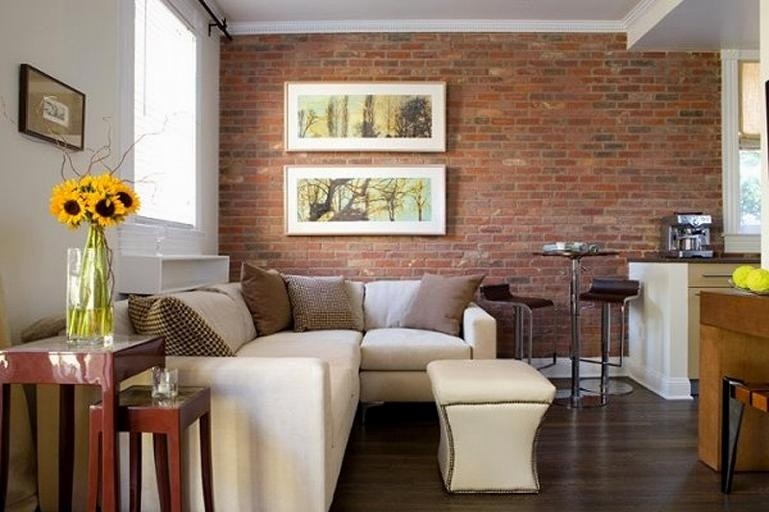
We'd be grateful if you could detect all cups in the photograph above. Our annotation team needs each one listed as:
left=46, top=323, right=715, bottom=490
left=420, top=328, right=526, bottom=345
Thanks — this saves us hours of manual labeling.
left=149, top=365, right=180, bottom=401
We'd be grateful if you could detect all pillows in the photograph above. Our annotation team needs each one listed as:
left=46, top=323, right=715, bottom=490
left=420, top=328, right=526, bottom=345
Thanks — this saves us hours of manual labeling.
left=399, top=267, right=487, bottom=340
left=128, top=293, right=238, bottom=357
left=240, top=262, right=366, bottom=338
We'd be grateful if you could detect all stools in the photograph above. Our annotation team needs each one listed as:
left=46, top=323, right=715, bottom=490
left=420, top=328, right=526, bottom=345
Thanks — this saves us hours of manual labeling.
left=569, top=276, right=641, bottom=398
left=722, top=376, right=769, bottom=493
left=423, top=359, right=557, bottom=496
left=480, top=282, right=559, bottom=372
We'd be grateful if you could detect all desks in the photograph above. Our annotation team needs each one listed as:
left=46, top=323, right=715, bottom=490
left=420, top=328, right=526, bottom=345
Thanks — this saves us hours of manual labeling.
left=698, top=289, right=769, bottom=474
left=0, top=336, right=215, bottom=511
left=531, top=251, right=622, bottom=408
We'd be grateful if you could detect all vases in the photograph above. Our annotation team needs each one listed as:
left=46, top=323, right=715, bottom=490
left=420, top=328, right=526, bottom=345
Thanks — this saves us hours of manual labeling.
left=63, top=247, right=115, bottom=349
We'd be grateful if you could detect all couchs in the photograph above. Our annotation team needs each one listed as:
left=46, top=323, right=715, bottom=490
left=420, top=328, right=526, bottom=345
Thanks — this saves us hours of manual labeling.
left=16, top=278, right=497, bottom=512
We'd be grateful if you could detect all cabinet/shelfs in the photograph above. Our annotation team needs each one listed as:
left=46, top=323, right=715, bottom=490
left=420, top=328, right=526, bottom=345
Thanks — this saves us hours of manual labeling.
left=119, top=253, right=231, bottom=296
left=689, top=264, right=762, bottom=395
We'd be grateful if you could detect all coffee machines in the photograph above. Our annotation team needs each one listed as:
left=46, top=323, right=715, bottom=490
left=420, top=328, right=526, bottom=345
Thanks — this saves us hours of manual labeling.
left=660, top=213, right=716, bottom=258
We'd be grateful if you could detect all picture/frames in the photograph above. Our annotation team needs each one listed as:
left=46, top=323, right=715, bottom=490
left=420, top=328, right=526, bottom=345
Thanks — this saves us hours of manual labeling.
left=17, top=62, right=86, bottom=154
left=281, top=79, right=449, bottom=238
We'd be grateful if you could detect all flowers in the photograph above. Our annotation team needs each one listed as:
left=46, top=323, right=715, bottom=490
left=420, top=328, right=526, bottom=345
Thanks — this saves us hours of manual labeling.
left=1, top=97, right=167, bottom=341
